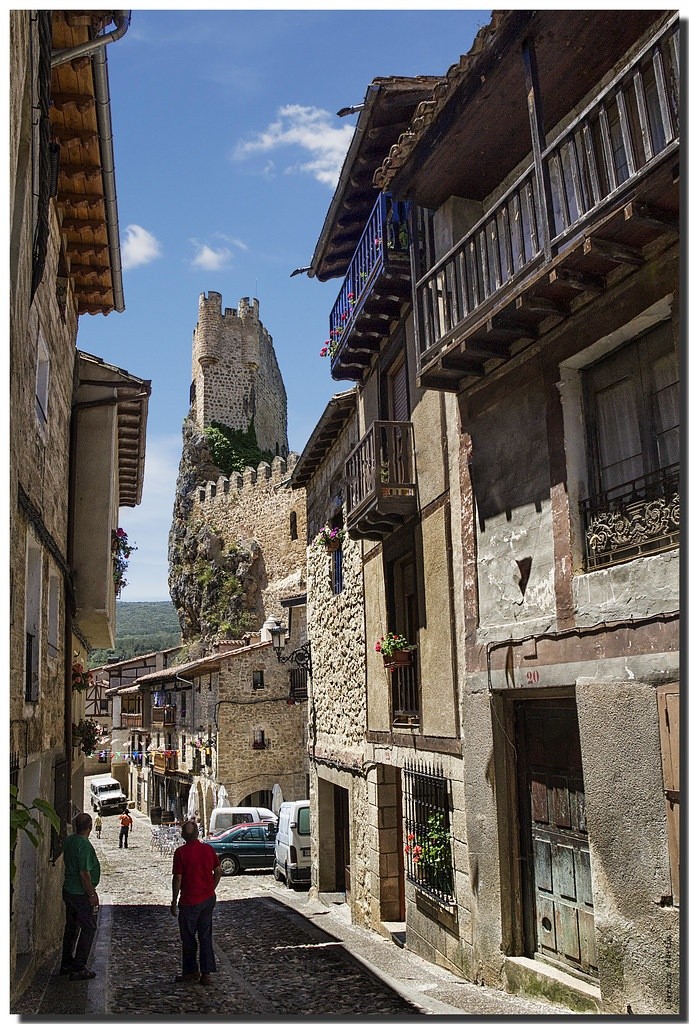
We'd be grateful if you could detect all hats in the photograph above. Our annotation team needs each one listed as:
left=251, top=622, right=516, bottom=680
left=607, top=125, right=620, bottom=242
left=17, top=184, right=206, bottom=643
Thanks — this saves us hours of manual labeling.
left=124, top=809, right=131, bottom=814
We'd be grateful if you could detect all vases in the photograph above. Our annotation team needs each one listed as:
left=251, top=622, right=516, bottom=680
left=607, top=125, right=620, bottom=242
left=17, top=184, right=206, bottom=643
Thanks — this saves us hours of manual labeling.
left=71, top=734, right=84, bottom=747
left=322, top=538, right=340, bottom=555
left=380, top=650, right=410, bottom=668
left=115, top=583, right=120, bottom=593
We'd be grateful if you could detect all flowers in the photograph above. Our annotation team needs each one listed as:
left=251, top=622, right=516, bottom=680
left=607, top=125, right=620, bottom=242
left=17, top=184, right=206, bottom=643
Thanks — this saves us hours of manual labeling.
left=71, top=714, right=100, bottom=755
left=320, top=220, right=410, bottom=361
left=405, top=812, right=451, bottom=877
left=72, top=662, right=94, bottom=691
left=310, top=526, right=345, bottom=552
left=376, top=633, right=408, bottom=657
left=109, top=525, right=137, bottom=593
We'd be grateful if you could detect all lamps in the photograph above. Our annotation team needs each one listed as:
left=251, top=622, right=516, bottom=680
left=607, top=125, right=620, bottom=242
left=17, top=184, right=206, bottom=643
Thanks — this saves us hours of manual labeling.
left=267, top=619, right=311, bottom=679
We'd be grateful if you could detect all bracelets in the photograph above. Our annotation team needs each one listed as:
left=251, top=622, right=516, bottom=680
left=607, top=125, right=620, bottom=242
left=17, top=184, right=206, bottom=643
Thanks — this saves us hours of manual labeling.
left=90, top=892, right=95, bottom=897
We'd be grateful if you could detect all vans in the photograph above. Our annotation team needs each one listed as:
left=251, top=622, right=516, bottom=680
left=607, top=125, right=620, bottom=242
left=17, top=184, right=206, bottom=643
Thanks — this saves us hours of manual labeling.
left=272, top=801, right=311, bottom=889
left=209, top=807, right=278, bottom=839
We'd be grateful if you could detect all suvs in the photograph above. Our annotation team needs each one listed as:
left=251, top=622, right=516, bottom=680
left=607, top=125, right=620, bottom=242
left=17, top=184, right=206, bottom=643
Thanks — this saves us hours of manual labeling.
left=90, top=777, right=128, bottom=817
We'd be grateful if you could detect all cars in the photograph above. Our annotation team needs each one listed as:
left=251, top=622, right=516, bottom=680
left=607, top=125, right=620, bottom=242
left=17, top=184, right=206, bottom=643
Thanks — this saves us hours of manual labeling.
left=203, top=826, right=279, bottom=876
left=205, top=822, right=277, bottom=841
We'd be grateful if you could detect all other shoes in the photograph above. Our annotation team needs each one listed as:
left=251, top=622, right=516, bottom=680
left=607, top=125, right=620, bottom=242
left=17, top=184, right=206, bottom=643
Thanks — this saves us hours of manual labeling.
left=70, top=970, right=97, bottom=982
left=175, top=971, right=200, bottom=982
left=200, top=973, right=215, bottom=986
left=59, top=965, right=74, bottom=975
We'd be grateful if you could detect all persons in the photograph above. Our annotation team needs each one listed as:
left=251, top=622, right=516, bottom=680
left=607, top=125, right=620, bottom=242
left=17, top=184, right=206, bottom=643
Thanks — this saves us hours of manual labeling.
left=94, top=812, right=102, bottom=839
left=170, top=821, right=222, bottom=983
left=59, top=814, right=100, bottom=980
left=118, top=809, right=133, bottom=848
